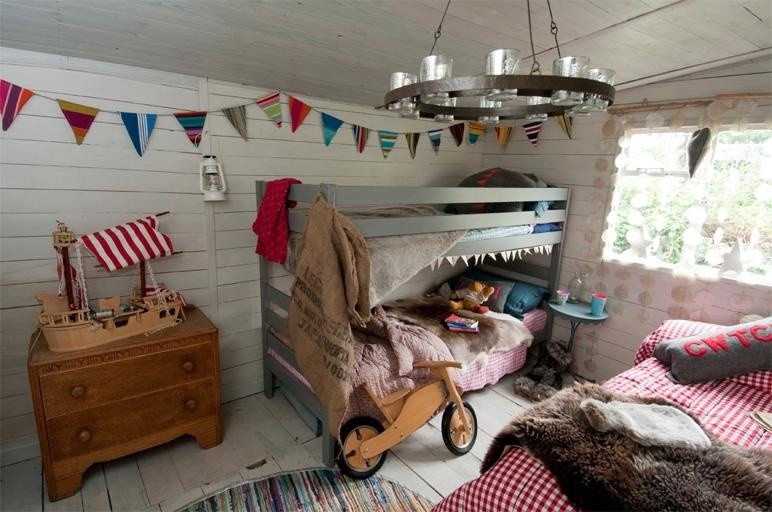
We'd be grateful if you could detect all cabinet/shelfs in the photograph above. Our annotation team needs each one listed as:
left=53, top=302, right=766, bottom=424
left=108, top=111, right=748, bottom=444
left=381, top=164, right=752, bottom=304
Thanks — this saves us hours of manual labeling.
left=26, top=304, right=223, bottom=503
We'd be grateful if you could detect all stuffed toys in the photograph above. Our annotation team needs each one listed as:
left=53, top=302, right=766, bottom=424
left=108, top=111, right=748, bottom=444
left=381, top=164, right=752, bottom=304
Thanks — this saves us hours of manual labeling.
left=447, top=278, right=496, bottom=317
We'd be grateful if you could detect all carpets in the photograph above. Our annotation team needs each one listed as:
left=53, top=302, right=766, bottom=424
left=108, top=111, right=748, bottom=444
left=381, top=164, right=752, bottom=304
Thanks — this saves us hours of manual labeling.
left=175, top=468, right=435, bottom=512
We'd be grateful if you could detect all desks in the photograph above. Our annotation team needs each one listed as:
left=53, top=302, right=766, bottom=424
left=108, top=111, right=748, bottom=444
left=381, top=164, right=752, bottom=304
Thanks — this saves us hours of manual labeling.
left=549, top=299, right=609, bottom=370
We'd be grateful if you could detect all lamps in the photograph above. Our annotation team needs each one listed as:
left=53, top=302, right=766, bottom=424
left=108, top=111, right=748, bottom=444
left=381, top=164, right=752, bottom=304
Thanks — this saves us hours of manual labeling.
left=200, top=153, right=226, bottom=202
left=375, top=0, right=616, bottom=129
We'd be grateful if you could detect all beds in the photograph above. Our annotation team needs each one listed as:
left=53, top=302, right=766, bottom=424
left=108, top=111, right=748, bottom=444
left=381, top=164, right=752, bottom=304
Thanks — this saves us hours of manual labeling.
left=429, top=356, right=772, bottom=512
left=255, top=176, right=571, bottom=468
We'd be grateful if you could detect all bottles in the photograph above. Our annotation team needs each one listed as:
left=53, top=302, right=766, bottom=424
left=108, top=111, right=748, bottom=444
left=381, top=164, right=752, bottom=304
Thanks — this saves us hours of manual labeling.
left=567, top=270, right=584, bottom=305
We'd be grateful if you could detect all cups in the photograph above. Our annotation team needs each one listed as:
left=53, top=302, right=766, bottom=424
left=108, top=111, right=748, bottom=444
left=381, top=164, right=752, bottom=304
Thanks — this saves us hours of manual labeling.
left=591, top=292, right=609, bottom=317
left=556, top=289, right=571, bottom=306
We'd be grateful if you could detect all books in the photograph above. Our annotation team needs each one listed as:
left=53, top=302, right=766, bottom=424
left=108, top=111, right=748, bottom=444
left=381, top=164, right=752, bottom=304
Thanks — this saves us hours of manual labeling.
left=444, top=313, right=479, bottom=328
left=446, top=322, right=477, bottom=330
left=747, top=408, right=772, bottom=433
left=448, top=326, right=480, bottom=333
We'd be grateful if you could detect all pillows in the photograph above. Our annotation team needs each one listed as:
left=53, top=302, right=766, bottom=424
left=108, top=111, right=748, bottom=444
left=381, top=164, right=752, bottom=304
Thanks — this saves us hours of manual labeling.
left=534, top=224, right=560, bottom=233
left=456, top=278, right=515, bottom=313
left=470, top=272, right=548, bottom=321
left=641, top=318, right=772, bottom=392
left=653, top=314, right=771, bottom=384
